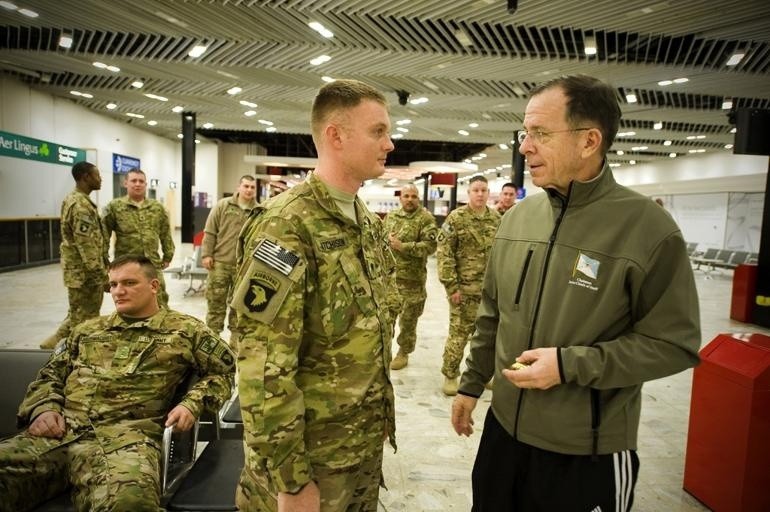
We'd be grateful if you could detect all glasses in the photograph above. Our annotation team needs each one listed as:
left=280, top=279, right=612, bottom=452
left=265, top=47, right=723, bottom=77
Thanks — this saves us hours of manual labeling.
left=518, top=128, right=589, bottom=145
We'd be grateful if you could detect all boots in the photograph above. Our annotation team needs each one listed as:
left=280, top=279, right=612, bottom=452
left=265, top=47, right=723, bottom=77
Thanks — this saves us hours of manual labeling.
left=40, top=335, right=57, bottom=350
left=391, top=346, right=408, bottom=369
left=229, top=332, right=238, bottom=354
left=443, top=376, right=458, bottom=395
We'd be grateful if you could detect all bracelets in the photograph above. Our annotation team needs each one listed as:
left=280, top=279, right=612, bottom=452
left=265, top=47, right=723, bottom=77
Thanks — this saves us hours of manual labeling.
left=284, top=481, right=308, bottom=495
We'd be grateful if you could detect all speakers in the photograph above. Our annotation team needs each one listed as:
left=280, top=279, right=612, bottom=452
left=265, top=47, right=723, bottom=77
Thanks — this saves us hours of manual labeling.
left=733, top=108, right=770, bottom=155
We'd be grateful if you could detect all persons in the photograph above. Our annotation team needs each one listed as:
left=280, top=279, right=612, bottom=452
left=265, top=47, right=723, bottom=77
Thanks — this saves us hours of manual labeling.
left=383, top=183, right=437, bottom=370
left=437, top=176, right=502, bottom=396
left=495, top=183, right=518, bottom=216
left=236, top=80, right=403, bottom=511
left=39, top=162, right=109, bottom=349
left=452, top=74, right=703, bottom=511
left=201, top=174, right=259, bottom=353
left=101, top=168, right=176, bottom=309
left=0, top=253, right=234, bottom=511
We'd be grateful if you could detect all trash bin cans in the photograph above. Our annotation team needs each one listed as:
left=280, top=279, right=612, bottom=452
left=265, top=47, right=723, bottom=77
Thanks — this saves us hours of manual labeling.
left=683, top=331, right=770, bottom=512
left=729, top=263, right=759, bottom=323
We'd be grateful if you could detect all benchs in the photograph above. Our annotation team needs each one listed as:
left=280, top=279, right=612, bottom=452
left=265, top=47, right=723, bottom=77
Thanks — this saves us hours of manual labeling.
left=1, top=346, right=203, bottom=503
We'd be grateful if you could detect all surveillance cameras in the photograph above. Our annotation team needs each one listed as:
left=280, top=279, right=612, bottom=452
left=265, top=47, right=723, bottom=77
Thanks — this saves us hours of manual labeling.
left=397, top=90, right=411, bottom=106
left=726, top=111, right=736, bottom=124
